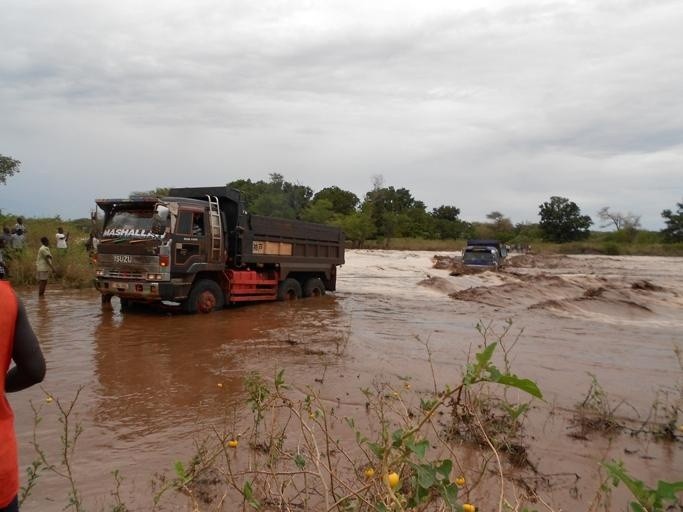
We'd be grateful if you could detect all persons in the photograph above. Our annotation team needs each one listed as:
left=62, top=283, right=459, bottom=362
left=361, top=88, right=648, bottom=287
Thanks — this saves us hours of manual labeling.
left=15, top=217, right=25, bottom=231
left=10, top=228, right=25, bottom=253
left=189, top=215, right=204, bottom=235
left=0, top=236, right=13, bottom=278
left=35, top=236, right=56, bottom=297
left=0, top=276, right=48, bottom=511
left=85, top=233, right=94, bottom=264
left=3, top=226, right=10, bottom=240
left=8, top=228, right=16, bottom=241
left=55, top=227, right=68, bottom=250
left=92, top=232, right=99, bottom=253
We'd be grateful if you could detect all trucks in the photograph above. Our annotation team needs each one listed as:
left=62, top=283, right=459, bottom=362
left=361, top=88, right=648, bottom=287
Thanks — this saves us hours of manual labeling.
left=87, top=182, right=347, bottom=317
left=458, top=237, right=509, bottom=273
